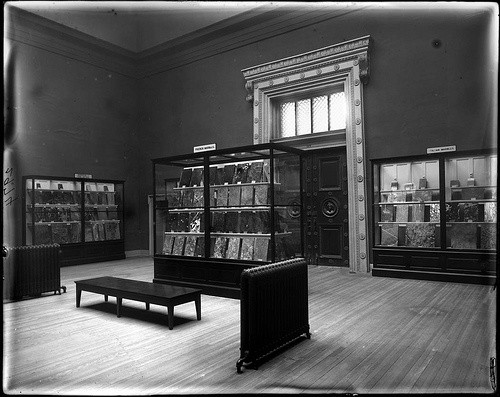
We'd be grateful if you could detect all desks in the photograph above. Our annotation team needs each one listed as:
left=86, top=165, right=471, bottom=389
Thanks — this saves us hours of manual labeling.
left=74, top=276, right=203, bottom=330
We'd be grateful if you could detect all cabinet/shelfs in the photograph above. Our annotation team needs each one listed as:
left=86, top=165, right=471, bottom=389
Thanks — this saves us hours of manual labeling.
left=150, top=139, right=306, bottom=300
left=367, top=147, right=498, bottom=285
left=22, top=175, right=126, bottom=267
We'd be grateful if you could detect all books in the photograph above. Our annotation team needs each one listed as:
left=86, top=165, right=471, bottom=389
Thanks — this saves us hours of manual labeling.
left=241, top=186, right=253, bottom=205
left=180, top=169, right=192, bottom=187
left=405, top=223, right=436, bottom=248
left=450, top=180, right=460, bottom=187
left=58, top=183, right=63, bottom=189
left=183, top=190, right=193, bottom=207
left=190, top=212, right=202, bottom=232
left=412, top=191, right=432, bottom=202
left=480, top=223, right=496, bottom=249
left=28, top=190, right=120, bottom=245
left=451, top=223, right=477, bottom=248
left=419, top=179, right=427, bottom=188
left=37, top=183, right=41, bottom=189
left=190, top=168, right=203, bottom=186
left=228, top=187, right=240, bottom=206
left=234, top=163, right=249, bottom=184
left=184, top=235, right=197, bottom=257
left=253, top=237, right=269, bottom=261
left=388, top=192, right=406, bottom=202
left=216, top=187, right=228, bottom=206
left=254, top=210, right=269, bottom=232
left=87, top=185, right=91, bottom=190
left=195, top=237, right=215, bottom=258
left=172, top=235, right=185, bottom=255
left=226, top=237, right=242, bottom=259
left=239, top=211, right=254, bottom=232
left=381, top=205, right=394, bottom=222
left=412, top=204, right=425, bottom=222
left=214, top=212, right=226, bottom=231
left=240, top=237, right=254, bottom=260
left=254, top=185, right=268, bottom=205
left=167, top=213, right=179, bottom=231
left=163, top=235, right=174, bottom=255
left=396, top=205, right=408, bottom=222
left=248, top=162, right=263, bottom=182
left=226, top=212, right=239, bottom=232
left=457, top=203, right=480, bottom=222
left=430, top=204, right=440, bottom=222
left=193, top=189, right=204, bottom=207
left=214, top=237, right=226, bottom=259
left=210, top=188, right=215, bottom=206
left=451, top=189, right=462, bottom=200
left=404, top=183, right=413, bottom=190
left=203, top=166, right=217, bottom=186
left=222, top=165, right=235, bottom=185
left=179, top=212, right=190, bottom=232
left=104, top=186, right=108, bottom=191
left=484, top=202, right=497, bottom=222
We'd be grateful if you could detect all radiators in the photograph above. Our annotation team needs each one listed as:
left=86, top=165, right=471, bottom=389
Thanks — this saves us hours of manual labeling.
left=4, top=243, right=66, bottom=302
left=237, top=258, right=311, bottom=373
